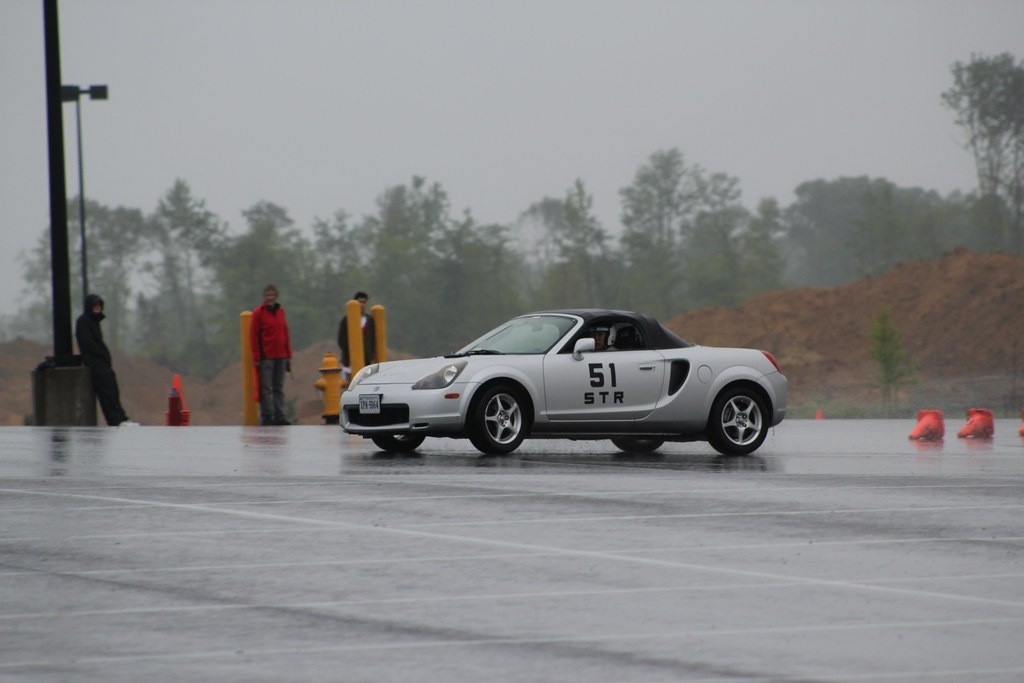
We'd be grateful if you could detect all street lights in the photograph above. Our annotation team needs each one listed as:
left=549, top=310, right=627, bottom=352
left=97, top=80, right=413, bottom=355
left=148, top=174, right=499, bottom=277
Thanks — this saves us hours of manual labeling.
left=59, top=84, right=108, bottom=303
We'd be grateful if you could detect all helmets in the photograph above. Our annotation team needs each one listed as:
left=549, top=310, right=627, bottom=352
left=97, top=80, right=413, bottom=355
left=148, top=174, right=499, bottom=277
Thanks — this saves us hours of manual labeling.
left=584, top=321, right=617, bottom=346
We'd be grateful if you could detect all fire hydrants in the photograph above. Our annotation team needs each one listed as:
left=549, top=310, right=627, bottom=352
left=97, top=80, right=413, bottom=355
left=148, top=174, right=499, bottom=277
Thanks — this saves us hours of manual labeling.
left=314, top=352, right=351, bottom=424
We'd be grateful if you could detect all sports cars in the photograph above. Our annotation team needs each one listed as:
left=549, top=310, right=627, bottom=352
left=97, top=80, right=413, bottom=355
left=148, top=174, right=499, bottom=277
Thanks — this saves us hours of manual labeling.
left=337, top=308, right=790, bottom=458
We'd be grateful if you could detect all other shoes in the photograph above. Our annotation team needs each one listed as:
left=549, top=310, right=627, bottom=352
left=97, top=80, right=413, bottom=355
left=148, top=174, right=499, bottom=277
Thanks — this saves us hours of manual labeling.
left=120, top=420, right=142, bottom=427
left=274, top=416, right=290, bottom=426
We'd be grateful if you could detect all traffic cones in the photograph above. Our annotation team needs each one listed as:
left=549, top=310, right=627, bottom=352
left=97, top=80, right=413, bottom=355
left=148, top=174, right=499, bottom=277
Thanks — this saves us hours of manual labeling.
left=816, top=407, right=823, bottom=419
left=958, top=406, right=996, bottom=438
left=1019, top=407, right=1024, bottom=438
left=907, top=407, right=945, bottom=442
left=165, top=373, right=191, bottom=427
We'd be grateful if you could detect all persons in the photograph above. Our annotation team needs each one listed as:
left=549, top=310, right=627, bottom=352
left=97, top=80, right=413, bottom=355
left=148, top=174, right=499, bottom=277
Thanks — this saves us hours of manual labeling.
left=76, top=294, right=140, bottom=427
left=249, top=284, right=294, bottom=425
left=584, top=320, right=619, bottom=352
left=338, top=292, right=375, bottom=378
